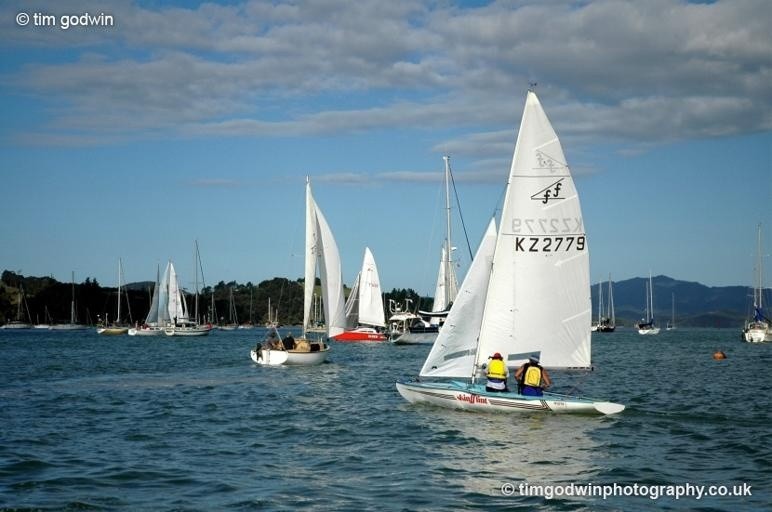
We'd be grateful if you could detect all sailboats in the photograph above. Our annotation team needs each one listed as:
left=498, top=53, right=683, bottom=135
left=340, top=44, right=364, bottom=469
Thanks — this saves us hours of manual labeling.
left=250, top=172, right=390, bottom=369
left=96, top=240, right=218, bottom=337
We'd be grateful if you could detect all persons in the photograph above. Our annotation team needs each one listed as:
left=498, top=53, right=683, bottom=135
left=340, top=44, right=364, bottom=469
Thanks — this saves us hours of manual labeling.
left=482, top=353, right=510, bottom=392
left=515, top=355, right=550, bottom=397
left=282, top=331, right=295, bottom=351
left=438, top=318, right=445, bottom=332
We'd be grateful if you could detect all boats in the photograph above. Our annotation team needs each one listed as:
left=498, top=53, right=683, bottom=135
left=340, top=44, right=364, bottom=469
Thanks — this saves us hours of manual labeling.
left=638, top=272, right=661, bottom=335
left=2, top=270, right=89, bottom=329
left=742, top=223, right=772, bottom=343
left=218, top=322, right=239, bottom=330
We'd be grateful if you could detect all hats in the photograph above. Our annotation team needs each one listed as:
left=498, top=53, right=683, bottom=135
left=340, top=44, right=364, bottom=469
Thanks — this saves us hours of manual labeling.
left=492, top=353, right=501, bottom=359
left=528, top=353, right=540, bottom=362
